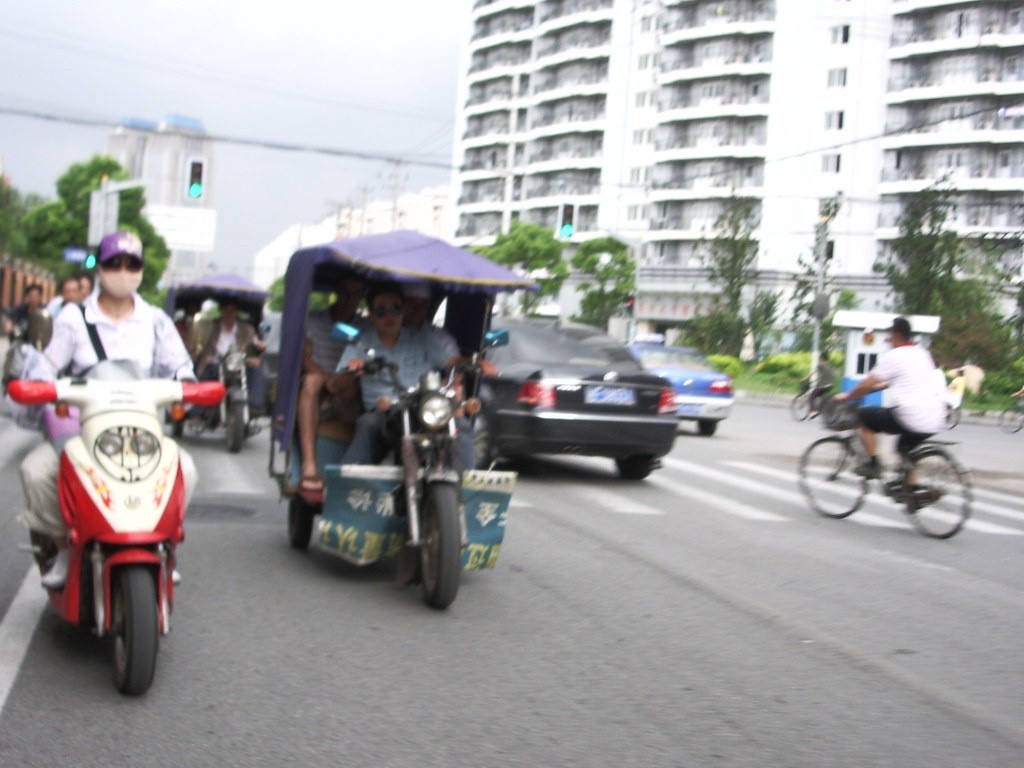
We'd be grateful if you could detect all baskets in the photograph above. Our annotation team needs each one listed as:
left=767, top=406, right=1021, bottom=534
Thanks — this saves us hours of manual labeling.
left=821, top=397, right=864, bottom=431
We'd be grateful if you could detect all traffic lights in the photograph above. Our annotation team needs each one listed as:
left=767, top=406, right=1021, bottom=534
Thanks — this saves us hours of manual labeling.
left=85, top=250, right=98, bottom=271
left=554, top=201, right=579, bottom=241
left=185, top=157, right=206, bottom=203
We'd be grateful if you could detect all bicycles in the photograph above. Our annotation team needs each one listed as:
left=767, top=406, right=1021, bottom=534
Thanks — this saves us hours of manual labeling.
left=800, top=396, right=973, bottom=540
left=791, top=379, right=834, bottom=423
left=946, top=395, right=1024, bottom=434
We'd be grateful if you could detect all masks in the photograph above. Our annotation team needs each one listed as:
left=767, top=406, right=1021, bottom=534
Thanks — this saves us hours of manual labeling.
left=98, top=267, right=144, bottom=297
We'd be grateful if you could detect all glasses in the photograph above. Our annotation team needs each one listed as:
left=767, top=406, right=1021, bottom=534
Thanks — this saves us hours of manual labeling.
left=374, top=304, right=405, bottom=318
left=101, top=255, right=142, bottom=271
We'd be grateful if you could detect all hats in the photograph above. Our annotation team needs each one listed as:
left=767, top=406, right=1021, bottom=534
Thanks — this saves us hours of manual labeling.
left=96, top=231, right=144, bottom=263
left=886, top=318, right=910, bottom=330
left=405, top=284, right=431, bottom=299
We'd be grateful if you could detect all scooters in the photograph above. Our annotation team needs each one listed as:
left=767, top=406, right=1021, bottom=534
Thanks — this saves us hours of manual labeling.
left=163, top=273, right=270, bottom=452
left=269, top=228, right=542, bottom=611
left=11, top=351, right=227, bottom=697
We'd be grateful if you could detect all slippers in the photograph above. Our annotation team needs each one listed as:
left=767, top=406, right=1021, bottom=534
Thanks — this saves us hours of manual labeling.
left=301, top=473, right=324, bottom=492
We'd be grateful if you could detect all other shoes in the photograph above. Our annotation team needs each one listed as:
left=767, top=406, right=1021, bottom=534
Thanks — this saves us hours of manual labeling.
left=172, top=570, right=182, bottom=586
left=41, top=548, right=68, bottom=590
left=851, top=462, right=883, bottom=478
left=809, top=411, right=820, bottom=419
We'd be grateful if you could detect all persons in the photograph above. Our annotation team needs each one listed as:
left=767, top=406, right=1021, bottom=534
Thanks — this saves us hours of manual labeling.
left=298, top=279, right=476, bottom=501
left=0, top=230, right=201, bottom=588
left=1011, top=386, right=1024, bottom=421
left=946, top=370, right=965, bottom=409
left=833, top=318, right=947, bottom=497
left=174, top=298, right=266, bottom=437
left=801, top=352, right=835, bottom=419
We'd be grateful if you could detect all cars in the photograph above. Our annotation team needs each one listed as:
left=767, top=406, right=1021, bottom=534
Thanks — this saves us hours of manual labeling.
left=479, top=317, right=679, bottom=482
left=629, top=341, right=735, bottom=438
